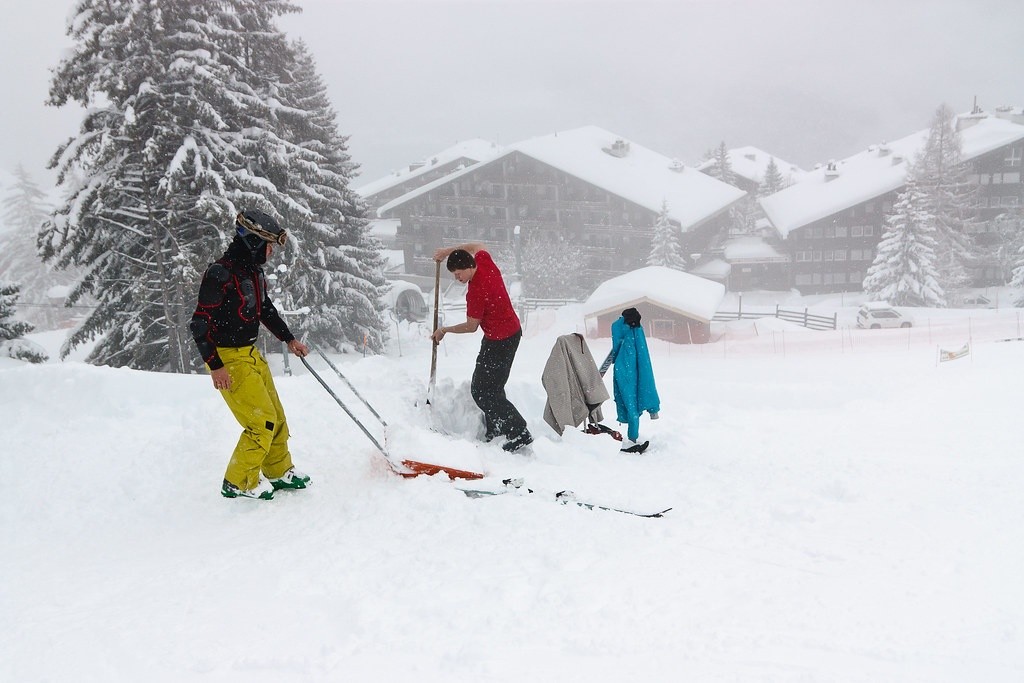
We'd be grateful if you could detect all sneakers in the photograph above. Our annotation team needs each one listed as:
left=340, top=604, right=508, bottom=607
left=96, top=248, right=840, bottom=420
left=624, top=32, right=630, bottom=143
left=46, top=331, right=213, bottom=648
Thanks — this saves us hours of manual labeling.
left=221, top=481, right=274, bottom=500
left=270, top=471, right=310, bottom=490
left=503, top=428, right=533, bottom=453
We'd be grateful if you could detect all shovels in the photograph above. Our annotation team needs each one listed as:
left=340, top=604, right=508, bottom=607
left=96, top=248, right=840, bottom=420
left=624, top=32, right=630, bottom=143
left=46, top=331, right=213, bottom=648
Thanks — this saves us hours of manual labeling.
left=423, top=259, right=445, bottom=408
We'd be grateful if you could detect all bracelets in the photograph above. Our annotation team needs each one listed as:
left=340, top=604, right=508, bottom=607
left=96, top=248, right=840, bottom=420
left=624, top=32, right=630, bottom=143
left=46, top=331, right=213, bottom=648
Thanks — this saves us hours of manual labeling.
left=440, top=328, right=446, bottom=335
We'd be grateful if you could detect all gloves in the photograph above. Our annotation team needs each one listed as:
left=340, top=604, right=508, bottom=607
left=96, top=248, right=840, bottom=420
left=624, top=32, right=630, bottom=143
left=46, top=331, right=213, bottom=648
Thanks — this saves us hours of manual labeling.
left=622, top=308, right=641, bottom=329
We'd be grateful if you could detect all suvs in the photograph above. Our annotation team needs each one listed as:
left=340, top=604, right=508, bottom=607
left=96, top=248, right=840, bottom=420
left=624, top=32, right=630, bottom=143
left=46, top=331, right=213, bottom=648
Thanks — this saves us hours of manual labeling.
left=856, top=302, right=914, bottom=328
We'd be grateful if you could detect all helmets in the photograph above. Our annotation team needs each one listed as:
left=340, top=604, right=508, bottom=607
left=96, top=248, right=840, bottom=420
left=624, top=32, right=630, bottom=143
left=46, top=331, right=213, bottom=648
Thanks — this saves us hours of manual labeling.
left=236, top=210, right=287, bottom=251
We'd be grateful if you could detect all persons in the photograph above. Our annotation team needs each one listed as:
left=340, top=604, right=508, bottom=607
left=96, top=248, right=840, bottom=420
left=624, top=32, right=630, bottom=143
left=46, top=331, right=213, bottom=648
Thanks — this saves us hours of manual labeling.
left=191, top=211, right=313, bottom=500
left=430, top=241, right=534, bottom=453
